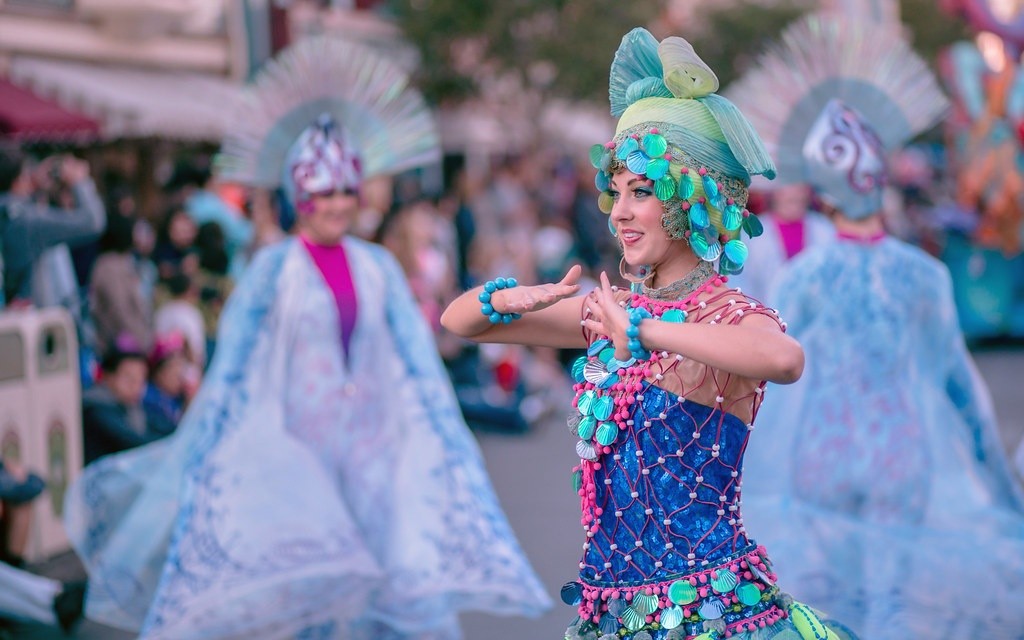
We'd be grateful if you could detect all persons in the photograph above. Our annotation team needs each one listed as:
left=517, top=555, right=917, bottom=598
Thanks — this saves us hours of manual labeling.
left=438, top=100, right=857, bottom=639
left=758, top=100, right=1024, bottom=638
left=0, top=121, right=1023, bottom=638
left=208, top=126, right=435, bottom=639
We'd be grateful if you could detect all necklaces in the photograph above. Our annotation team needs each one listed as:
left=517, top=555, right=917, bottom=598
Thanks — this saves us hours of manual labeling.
left=639, top=260, right=718, bottom=310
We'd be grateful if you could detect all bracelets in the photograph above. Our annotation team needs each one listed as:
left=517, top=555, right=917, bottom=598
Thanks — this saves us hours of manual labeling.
left=625, top=308, right=654, bottom=360
left=478, top=276, right=522, bottom=324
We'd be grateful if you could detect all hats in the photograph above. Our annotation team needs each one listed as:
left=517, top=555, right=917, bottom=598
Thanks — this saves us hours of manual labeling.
left=589, top=26, right=778, bottom=274
